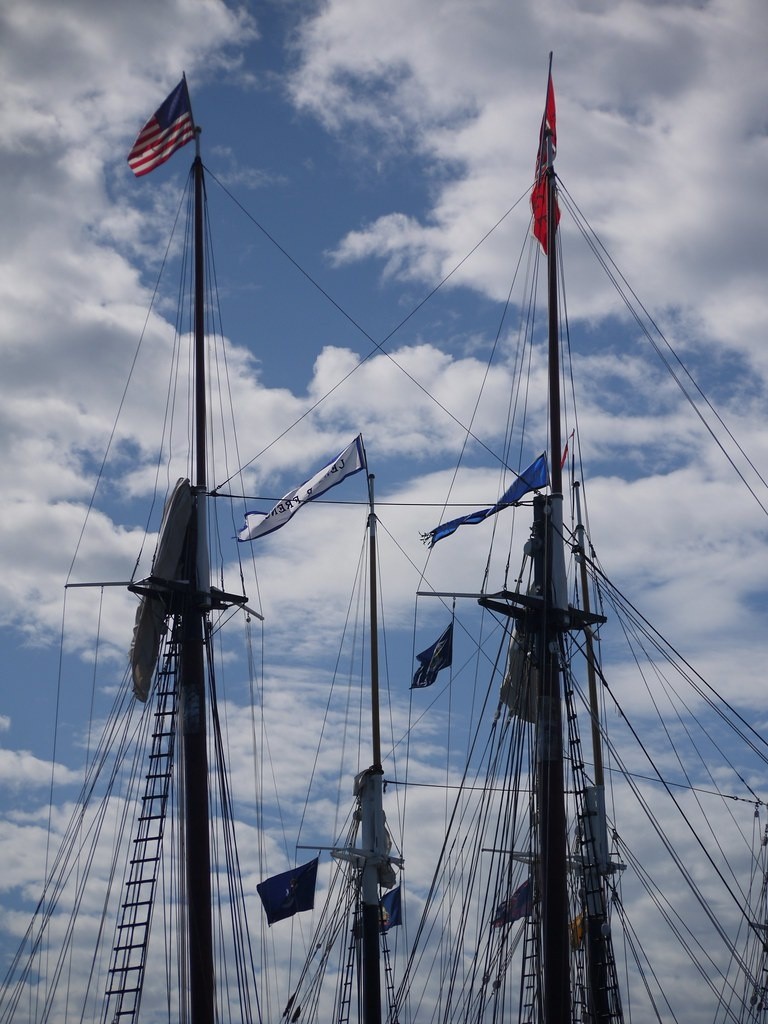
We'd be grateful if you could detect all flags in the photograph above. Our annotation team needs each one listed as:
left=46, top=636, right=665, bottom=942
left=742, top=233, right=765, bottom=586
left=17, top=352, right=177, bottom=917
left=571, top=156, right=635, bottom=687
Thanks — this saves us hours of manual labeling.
left=125, top=76, right=197, bottom=177
left=408, top=620, right=454, bottom=689
left=528, top=72, right=560, bottom=253
left=491, top=874, right=534, bottom=927
left=427, top=450, right=551, bottom=549
left=352, top=886, right=403, bottom=941
left=570, top=909, right=585, bottom=952
left=235, top=429, right=367, bottom=541
left=256, top=856, right=319, bottom=925
